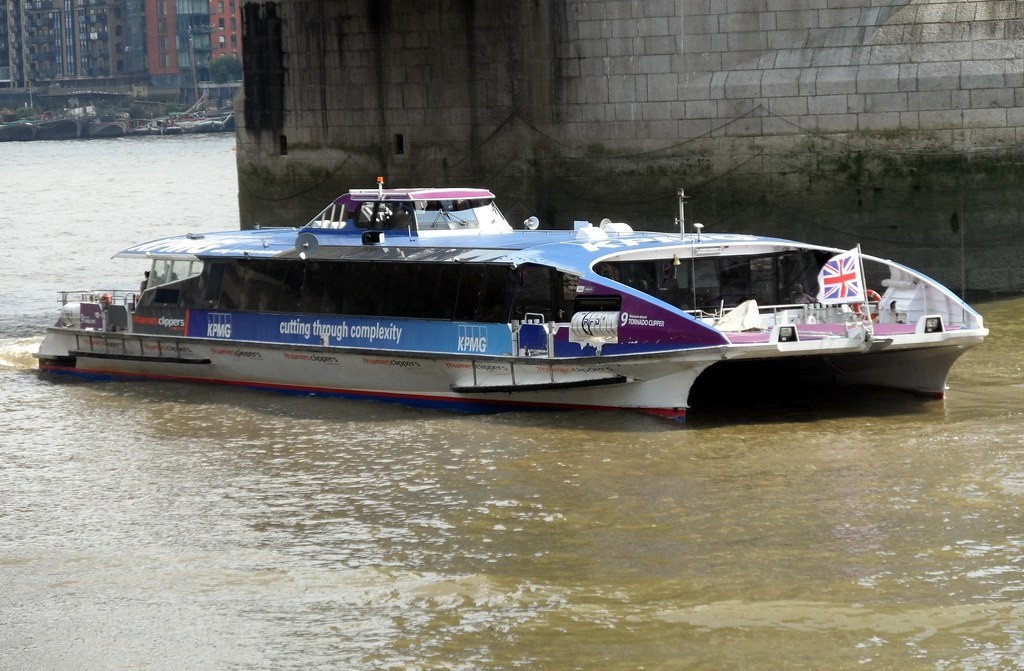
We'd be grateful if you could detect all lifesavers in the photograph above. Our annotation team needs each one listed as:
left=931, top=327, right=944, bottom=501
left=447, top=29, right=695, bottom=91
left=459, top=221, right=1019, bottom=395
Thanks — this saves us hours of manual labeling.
left=99, top=293, right=112, bottom=305
left=852, top=289, right=883, bottom=322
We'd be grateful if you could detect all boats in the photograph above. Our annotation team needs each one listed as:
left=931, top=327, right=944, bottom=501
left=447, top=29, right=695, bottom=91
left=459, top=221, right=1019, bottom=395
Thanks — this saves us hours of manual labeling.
left=0, top=113, right=235, bottom=141
left=28, top=176, right=990, bottom=425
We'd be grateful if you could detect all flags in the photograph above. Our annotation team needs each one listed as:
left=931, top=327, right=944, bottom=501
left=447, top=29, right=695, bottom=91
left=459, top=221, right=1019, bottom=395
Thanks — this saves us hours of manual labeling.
left=816, top=246, right=865, bottom=304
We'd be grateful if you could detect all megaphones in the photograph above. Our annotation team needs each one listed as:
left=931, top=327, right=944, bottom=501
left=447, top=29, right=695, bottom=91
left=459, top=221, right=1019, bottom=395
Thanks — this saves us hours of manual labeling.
left=524, top=216, right=539, bottom=230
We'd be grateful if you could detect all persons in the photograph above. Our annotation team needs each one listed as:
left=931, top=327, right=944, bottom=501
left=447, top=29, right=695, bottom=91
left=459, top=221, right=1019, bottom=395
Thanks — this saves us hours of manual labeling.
left=140, top=264, right=180, bottom=294
